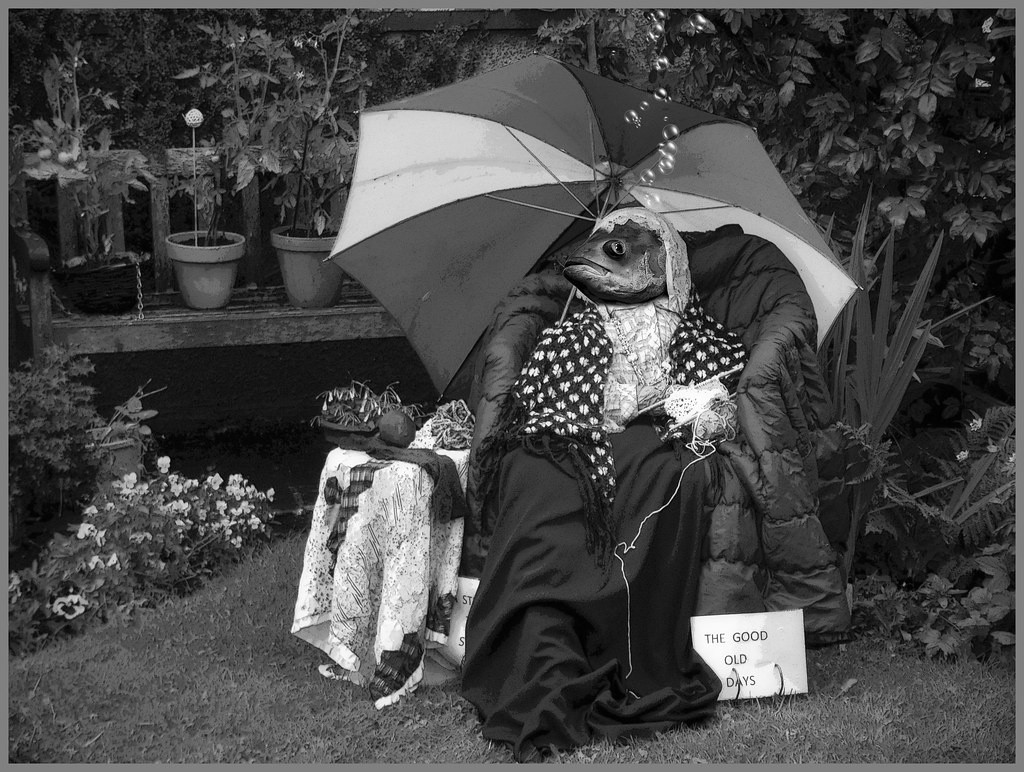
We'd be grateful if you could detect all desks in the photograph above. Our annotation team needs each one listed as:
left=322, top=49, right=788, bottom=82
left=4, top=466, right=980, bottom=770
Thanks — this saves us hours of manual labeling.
left=297, top=439, right=477, bottom=694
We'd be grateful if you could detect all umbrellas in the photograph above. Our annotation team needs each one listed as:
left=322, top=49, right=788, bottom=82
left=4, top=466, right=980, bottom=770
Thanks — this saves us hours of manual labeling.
left=319, top=50, right=864, bottom=415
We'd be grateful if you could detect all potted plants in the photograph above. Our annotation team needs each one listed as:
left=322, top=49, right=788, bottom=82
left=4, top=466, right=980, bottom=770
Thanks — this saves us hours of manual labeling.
left=267, top=10, right=383, bottom=310
left=25, top=42, right=155, bottom=313
left=168, top=27, right=276, bottom=306
left=79, top=420, right=144, bottom=490
